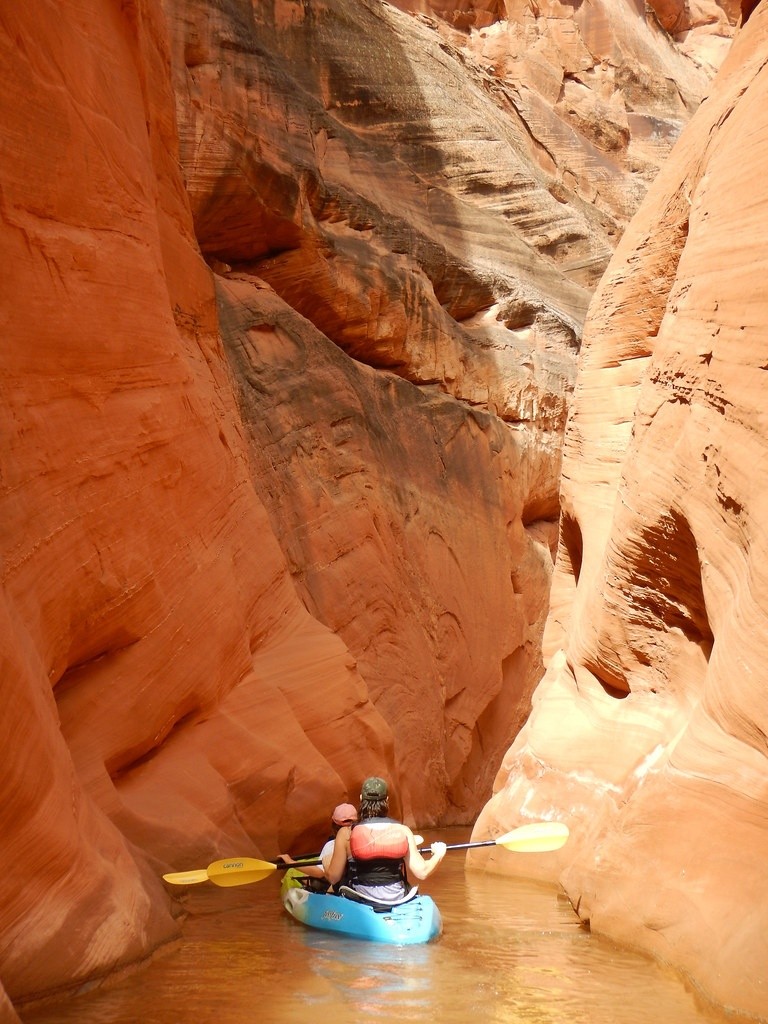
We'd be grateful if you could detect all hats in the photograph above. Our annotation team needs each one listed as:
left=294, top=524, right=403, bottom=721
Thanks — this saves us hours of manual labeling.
left=331, top=801, right=358, bottom=827
left=361, top=777, right=388, bottom=801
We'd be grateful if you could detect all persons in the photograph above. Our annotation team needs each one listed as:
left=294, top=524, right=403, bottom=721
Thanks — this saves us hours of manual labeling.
left=322, top=778, right=447, bottom=900
left=277, top=803, right=358, bottom=877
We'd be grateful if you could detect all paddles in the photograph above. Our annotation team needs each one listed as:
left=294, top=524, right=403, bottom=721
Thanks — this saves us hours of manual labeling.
left=160, top=832, right=425, bottom=885
left=204, top=819, right=571, bottom=889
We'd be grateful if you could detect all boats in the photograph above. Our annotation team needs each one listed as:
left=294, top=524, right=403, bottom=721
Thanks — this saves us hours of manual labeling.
left=280, top=858, right=443, bottom=945
